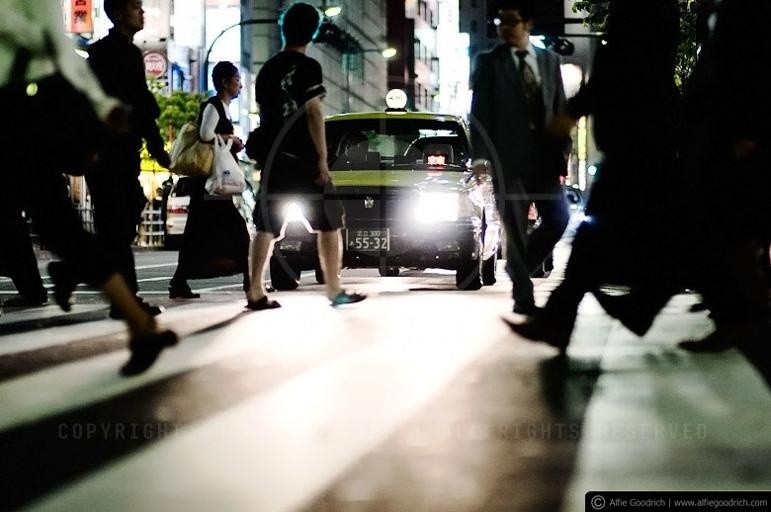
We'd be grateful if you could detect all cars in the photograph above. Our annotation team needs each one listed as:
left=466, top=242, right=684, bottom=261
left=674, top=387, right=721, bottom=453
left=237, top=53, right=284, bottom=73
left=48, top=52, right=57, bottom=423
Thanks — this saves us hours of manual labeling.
left=562, top=179, right=585, bottom=212
left=269, top=89, right=503, bottom=290
left=164, top=177, right=256, bottom=235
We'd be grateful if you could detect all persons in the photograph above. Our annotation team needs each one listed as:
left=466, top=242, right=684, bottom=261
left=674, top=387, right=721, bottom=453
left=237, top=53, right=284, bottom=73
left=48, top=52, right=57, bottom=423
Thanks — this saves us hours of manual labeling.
left=168, top=58, right=278, bottom=302
left=244, top=3, right=365, bottom=311
left=336, top=131, right=372, bottom=170
left=1, top=1, right=181, bottom=381
left=464, top=1, right=771, bottom=356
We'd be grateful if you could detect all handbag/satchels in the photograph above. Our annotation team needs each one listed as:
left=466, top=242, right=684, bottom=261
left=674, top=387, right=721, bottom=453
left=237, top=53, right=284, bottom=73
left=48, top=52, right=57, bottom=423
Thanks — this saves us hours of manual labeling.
left=3, top=72, right=100, bottom=177
left=170, top=122, right=214, bottom=176
left=246, top=128, right=304, bottom=168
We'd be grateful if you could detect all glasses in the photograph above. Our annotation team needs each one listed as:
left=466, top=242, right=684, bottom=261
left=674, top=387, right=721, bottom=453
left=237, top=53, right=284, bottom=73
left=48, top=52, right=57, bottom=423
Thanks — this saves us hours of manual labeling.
left=497, top=17, right=523, bottom=28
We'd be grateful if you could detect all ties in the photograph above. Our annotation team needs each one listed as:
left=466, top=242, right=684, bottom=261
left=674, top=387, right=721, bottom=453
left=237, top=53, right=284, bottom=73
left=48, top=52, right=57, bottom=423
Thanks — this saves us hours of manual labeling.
left=516, top=50, right=537, bottom=96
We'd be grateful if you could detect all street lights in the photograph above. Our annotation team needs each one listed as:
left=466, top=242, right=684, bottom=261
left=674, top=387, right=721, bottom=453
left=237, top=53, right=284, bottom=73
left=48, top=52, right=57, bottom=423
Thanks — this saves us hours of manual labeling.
left=203, top=7, right=343, bottom=90
left=342, top=48, right=397, bottom=112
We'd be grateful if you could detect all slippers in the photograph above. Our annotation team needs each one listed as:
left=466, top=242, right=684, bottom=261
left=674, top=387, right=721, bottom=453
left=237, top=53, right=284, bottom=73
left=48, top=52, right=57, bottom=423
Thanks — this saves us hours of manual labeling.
left=246, top=297, right=283, bottom=310
left=329, top=290, right=368, bottom=307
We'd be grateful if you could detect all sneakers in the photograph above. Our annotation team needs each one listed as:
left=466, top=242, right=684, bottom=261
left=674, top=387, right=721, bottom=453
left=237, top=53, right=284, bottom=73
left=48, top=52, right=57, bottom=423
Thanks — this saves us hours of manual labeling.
left=502, top=264, right=735, bottom=354
left=2, top=260, right=201, bottom=376
